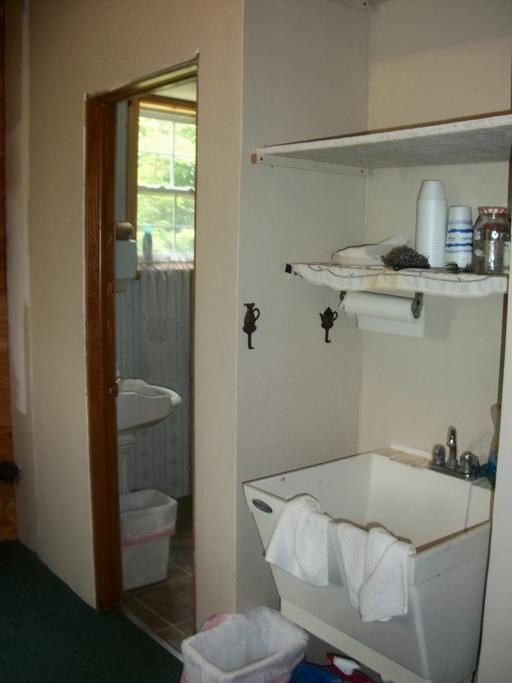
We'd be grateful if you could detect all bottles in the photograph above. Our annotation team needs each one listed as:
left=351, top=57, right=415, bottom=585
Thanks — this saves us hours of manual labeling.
left=473, top=204, right=512, bottom=275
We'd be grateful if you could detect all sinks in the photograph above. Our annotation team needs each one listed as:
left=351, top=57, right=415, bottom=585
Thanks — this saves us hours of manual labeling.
left=116, top=377, right=183, bottom=433
left=241, top=442, right=495, bottom=683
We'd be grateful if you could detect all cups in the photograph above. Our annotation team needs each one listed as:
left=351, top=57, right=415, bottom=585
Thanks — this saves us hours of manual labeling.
left=415, top=180, right=473, bottom=272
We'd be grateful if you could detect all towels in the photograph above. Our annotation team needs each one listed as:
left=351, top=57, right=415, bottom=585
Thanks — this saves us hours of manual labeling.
left=263, top=491, right=417, bottom=624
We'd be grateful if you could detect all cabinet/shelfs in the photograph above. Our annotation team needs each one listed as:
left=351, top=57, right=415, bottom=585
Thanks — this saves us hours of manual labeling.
left=252, top=107, right=512, bottom=302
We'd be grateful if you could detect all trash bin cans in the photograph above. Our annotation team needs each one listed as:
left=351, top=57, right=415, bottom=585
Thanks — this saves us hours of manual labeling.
left=119, top=489, right=178, bottom=591
left=179, top=605, right=310, bottom=683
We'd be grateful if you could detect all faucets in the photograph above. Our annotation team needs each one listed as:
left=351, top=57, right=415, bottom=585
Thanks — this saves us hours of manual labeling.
left=445, top=423, right=460, bottom=472
